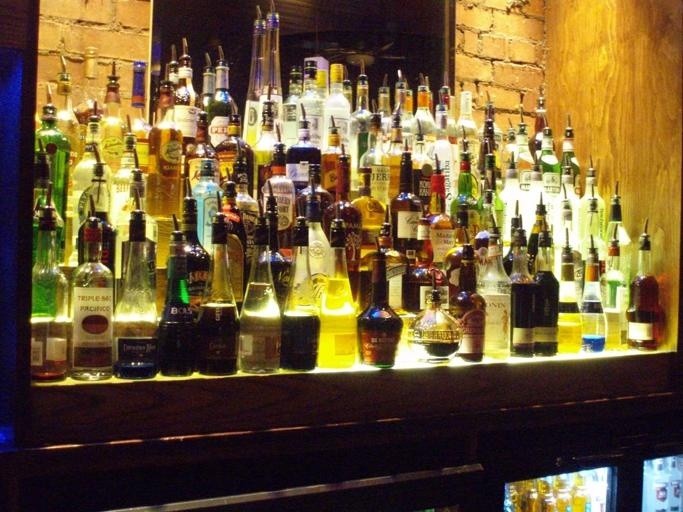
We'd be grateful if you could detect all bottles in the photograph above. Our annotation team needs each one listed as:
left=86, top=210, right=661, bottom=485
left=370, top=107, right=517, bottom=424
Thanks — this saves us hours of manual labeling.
left=507, top=457, right=682, bottom=512
left=28, top=1, right=669, bottom=379
